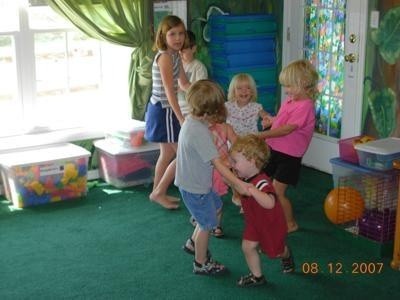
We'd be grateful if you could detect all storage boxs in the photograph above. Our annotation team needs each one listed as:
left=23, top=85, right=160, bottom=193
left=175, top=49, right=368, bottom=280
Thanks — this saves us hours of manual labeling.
left=0, top=143, right=92, bottom=209
left=354, top=137, right=400, bottom=172
left=330, top=157, right=399, bottom=211
left=92, top=139, right=160, bottom=189
left=105, top=121, right=147, bottom=148
left=337, top=136, right=379, bottom=165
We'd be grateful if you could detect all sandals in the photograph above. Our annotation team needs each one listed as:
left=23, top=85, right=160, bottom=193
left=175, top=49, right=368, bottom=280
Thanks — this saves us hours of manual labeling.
left=182, top=237, right=228, bottom=275
left=189, top=215, right=225, bottom=237
left=282, top=247, right=295, bottom=273
left=236, top=274, right=266, bottom=287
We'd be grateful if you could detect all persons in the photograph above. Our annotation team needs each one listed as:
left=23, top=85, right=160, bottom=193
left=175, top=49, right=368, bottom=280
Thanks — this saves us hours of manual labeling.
left=176, top=31, right=208, bottom=120
left=224, top=73, right=273, bottom=213
left=146, top=17, right=191, bottom=209
left=223, top=133, right=294, bottom=289
left=174, top=79, right=253, bottom=275
left=190, top=104, right=238, bottom=236
left=253, top=60, right=316, bottom=233
